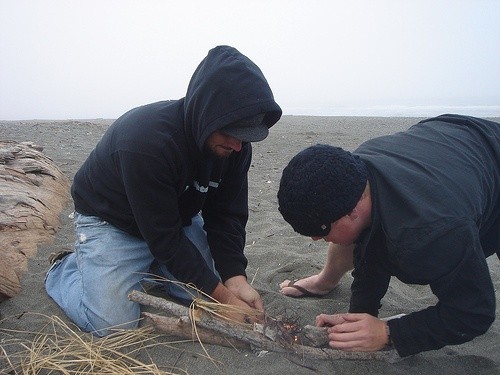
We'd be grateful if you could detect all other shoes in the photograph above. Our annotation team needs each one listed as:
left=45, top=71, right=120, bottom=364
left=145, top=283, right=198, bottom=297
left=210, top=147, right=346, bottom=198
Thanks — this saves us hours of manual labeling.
left=48, top=250, right=74, bottom=265
left=147, top=263, right=166, bottom=282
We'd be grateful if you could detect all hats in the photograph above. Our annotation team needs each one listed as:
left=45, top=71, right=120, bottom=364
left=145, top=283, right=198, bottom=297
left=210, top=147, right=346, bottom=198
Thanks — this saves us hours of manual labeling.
left=277, top=144, right=368, bottom=236
left=219, top=113, right=269, bottom=142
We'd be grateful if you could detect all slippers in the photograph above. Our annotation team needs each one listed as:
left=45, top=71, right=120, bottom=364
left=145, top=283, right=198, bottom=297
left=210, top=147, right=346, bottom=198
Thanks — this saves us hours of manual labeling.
left=278, top=275, right=338, bottom=298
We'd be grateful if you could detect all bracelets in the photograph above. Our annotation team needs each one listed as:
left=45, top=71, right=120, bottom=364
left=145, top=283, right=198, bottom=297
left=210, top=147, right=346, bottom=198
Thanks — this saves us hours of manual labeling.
left=385, top=321, right=392, bottom=347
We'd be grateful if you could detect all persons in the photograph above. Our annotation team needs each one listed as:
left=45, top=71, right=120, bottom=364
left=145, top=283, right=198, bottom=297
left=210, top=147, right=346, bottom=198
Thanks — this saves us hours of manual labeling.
left=42, top=45, right=282, bottom=338
left=277, top=114, right=500, bottom=358
